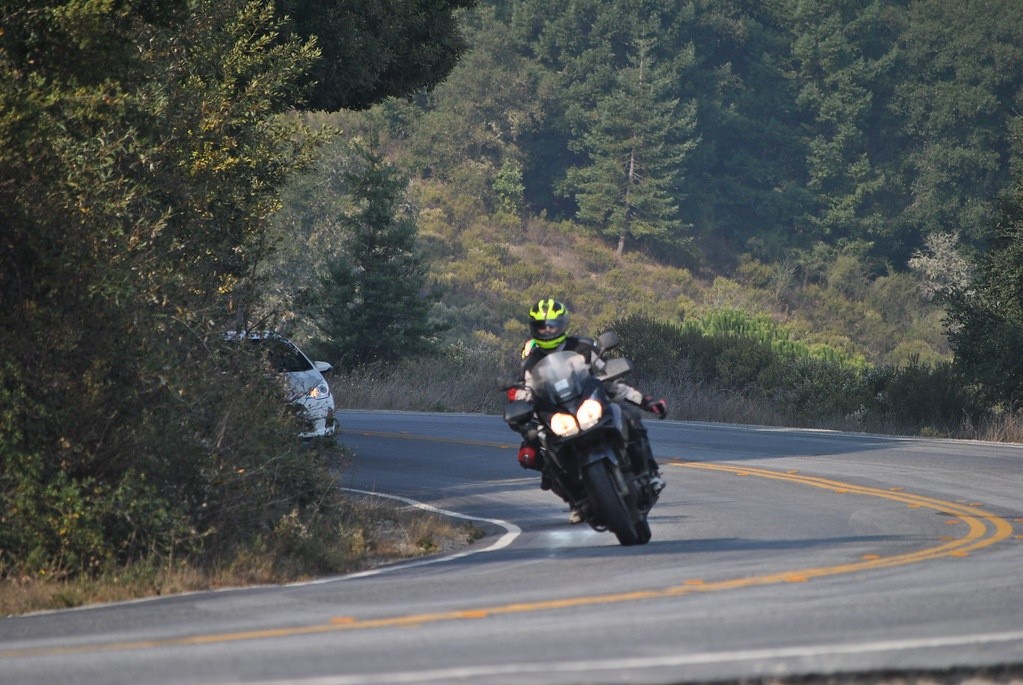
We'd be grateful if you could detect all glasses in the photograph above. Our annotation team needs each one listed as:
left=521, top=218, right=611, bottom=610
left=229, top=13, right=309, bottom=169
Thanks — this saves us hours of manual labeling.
left=533, top=321, right=561, bottom=330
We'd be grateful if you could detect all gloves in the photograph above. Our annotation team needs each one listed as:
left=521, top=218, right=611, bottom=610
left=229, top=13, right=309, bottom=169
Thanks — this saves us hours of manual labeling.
left=641, top=394, right=669, bottom=420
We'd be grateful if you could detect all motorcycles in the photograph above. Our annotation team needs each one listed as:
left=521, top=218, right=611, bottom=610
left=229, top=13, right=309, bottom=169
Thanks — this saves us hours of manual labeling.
left=493, top=331, right=666, bottom=548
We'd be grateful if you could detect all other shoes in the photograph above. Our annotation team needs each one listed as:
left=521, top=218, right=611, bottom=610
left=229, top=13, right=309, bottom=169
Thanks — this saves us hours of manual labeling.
left=567, top=509, right=584, bottom=525
left=649, top=475, right=666, bottom=493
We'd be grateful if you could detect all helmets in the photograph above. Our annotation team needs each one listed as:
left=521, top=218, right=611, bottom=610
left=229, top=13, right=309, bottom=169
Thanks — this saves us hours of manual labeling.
left=520, top=338, right=539, bottom=360
left=528, top=298, right=571, bottom=349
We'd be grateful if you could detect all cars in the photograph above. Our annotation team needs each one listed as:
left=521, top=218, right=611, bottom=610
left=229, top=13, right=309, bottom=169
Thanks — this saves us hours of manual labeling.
left=227, top=332, right=336, bottom=444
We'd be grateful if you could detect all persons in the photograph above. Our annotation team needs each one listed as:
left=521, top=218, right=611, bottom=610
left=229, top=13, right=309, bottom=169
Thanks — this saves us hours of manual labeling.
left=508, top=299, right=669, bottom=525
